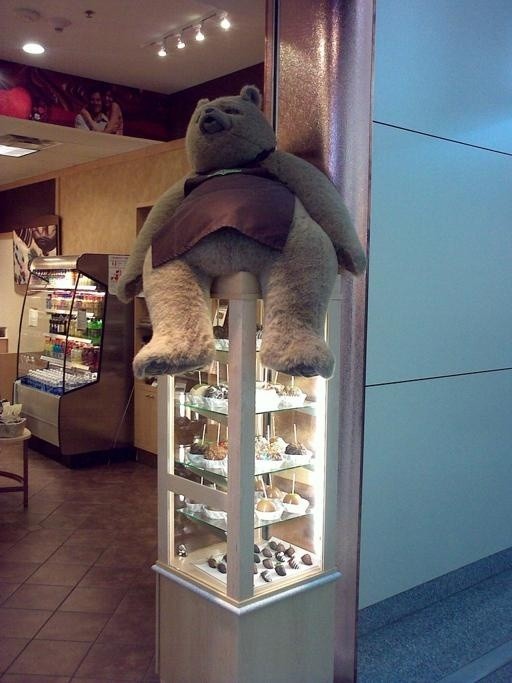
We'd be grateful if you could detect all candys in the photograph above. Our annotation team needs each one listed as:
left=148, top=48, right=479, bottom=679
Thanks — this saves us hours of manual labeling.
left=186, top=302, right=314, bottom=521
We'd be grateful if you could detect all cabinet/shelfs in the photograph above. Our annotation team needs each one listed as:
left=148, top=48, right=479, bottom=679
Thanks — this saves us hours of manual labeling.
left=152, top=271, right=342, bottom=683
left=9, top=253, right=136, bottom=469
left=133, top=289, right=157, bottom=461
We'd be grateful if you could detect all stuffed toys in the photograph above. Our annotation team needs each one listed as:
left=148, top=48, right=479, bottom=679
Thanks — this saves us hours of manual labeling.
left=112, top=81, right=369, bottom=379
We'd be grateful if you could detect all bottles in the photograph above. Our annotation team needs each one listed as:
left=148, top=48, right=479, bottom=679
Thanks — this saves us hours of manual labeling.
left=26, top=288, right=105, bottom=395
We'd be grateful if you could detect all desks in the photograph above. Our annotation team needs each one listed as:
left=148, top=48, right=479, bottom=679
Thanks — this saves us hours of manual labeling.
left=0, top=428, right=32, bottom=509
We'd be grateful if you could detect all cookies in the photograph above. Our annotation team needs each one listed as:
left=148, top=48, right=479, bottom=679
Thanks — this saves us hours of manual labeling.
left=208, top=540, right=312, bottom=581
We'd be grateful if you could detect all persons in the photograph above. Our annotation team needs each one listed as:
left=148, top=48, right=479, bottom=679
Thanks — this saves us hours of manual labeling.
left=74, top=86, right=124, bottom=135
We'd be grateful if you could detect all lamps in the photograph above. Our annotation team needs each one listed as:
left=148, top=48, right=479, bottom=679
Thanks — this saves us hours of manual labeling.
left=140, top=8, right=231, bottom=56
